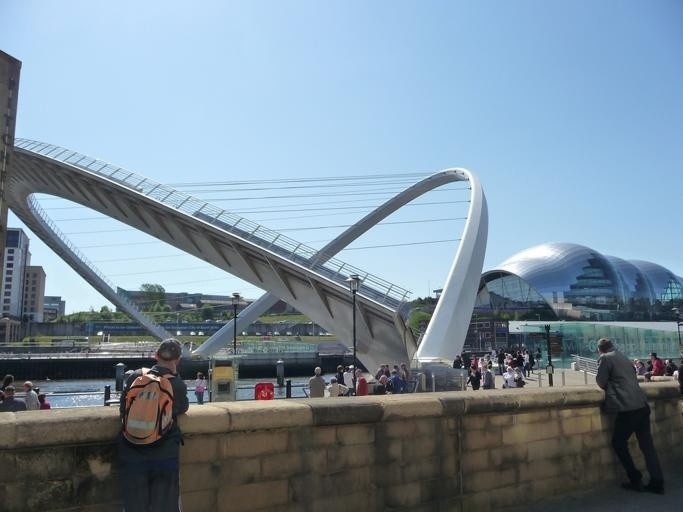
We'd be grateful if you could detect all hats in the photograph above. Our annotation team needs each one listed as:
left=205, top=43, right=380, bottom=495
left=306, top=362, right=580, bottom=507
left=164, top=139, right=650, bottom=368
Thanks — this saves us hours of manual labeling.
left=5, top=386, right=16, bottom=391
left=157, top=338, right=182, bottom=360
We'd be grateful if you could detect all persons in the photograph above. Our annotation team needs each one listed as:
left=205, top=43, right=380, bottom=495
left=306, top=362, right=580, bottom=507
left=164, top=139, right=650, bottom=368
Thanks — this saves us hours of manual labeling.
left=115, top=338, right=190, bottom=511
left=37, top=394, right=50, bottom=410
left=595, top=337, right=666, bottom=497
left=194, top=371, right=205, bottom=402
left=453, top=345, right=543, bottom=391
left=0, top=385, right=27, bottom=413
left=0, top=374, right=15, bottom=390
left=309, top=366, right=326, bottom=396
left=22, top=380, right=41, bottom=411
left=632, top=350, right=682, bottom=397
left=326, top=363, right=410, bottom=396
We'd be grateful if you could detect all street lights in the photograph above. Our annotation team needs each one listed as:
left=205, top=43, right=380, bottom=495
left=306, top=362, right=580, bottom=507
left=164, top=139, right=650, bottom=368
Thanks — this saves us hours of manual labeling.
left=231, top=292, right=240, bottom=355
left=343, top=274, right=366, bottom=396
left=671, top=307, right=681, bottom=345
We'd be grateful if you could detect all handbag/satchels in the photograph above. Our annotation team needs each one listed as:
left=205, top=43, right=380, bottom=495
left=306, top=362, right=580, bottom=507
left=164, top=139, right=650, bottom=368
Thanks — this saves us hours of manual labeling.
left=196, top=386, right=204, bottom=392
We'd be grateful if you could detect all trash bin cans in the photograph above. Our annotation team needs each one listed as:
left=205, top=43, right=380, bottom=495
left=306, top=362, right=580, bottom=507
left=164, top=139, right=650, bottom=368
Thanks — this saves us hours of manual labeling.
left=255, top=383, right=274, bottom=399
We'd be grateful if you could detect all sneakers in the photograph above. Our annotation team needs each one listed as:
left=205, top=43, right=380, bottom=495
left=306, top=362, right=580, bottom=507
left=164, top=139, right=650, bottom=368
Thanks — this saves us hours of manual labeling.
left=645, top=480, right=664, bottom=494
left=623, top=479, right=645, bottom=492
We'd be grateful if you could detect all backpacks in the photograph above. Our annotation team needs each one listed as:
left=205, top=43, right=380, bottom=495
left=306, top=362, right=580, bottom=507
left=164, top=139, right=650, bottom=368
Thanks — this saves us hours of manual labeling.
left=120, top=367, right=176, bottom=450
